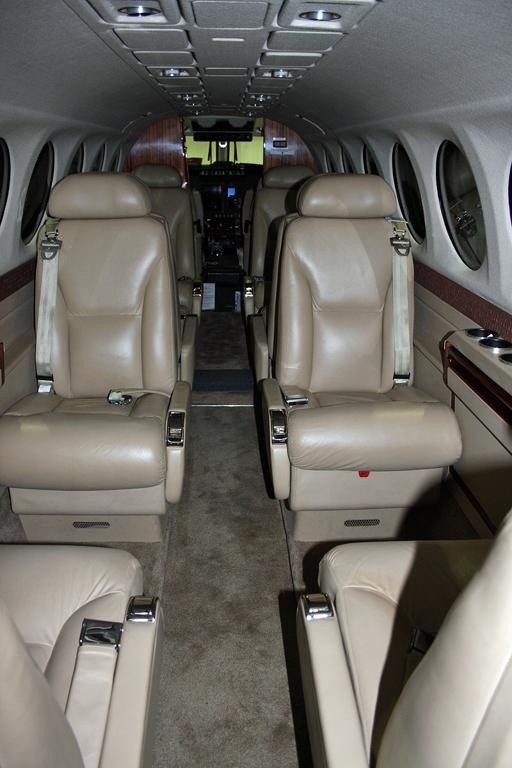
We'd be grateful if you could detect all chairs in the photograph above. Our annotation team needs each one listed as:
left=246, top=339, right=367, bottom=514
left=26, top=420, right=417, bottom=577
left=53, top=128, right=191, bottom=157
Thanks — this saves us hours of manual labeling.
left=239, top=167, right=512, bottom=768
left=0, top=133, right=204, bottom=768
